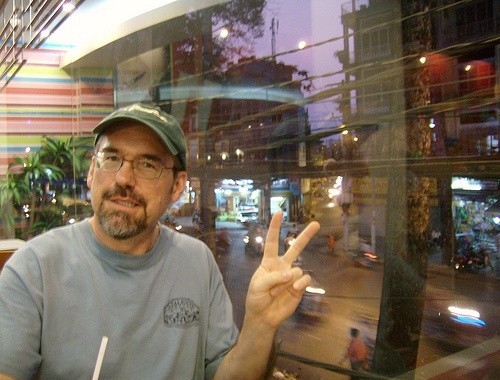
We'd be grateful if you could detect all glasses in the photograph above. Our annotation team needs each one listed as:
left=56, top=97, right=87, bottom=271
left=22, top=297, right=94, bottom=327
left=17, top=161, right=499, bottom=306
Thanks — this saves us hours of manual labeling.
left=94, top=152, right=175, bottom=179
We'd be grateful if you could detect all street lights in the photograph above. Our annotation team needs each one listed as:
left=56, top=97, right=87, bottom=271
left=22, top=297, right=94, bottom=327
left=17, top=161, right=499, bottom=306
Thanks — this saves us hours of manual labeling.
left=200, top=28, right=229, bottom=251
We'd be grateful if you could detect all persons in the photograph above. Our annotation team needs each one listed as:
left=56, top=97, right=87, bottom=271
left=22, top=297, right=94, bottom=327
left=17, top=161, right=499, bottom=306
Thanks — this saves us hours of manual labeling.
left=0, top=105, right=321, bottom=380
left=339, top=327, right=369, bottom=380
left=272, top=366, right=298, bottom=380
left=165, top=208, right=442, bottom=265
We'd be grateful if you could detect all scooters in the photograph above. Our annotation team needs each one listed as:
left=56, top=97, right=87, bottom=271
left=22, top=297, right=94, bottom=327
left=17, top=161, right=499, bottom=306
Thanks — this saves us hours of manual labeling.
left=352, top=250, right=377, bottom=269
left=215, top=235, right=232, bottom=252
left=454, top=246, right=494, bottom=275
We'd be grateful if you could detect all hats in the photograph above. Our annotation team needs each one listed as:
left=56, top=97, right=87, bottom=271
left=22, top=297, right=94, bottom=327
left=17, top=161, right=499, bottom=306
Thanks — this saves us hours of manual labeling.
left=93, top=103, right=185, bottom=169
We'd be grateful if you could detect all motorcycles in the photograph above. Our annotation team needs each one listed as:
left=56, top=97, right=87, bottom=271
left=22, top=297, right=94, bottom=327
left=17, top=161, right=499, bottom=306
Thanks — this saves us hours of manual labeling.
left=283, top=233, right=298, bottom=250
left=245, top=233, right=265, bottom=256
